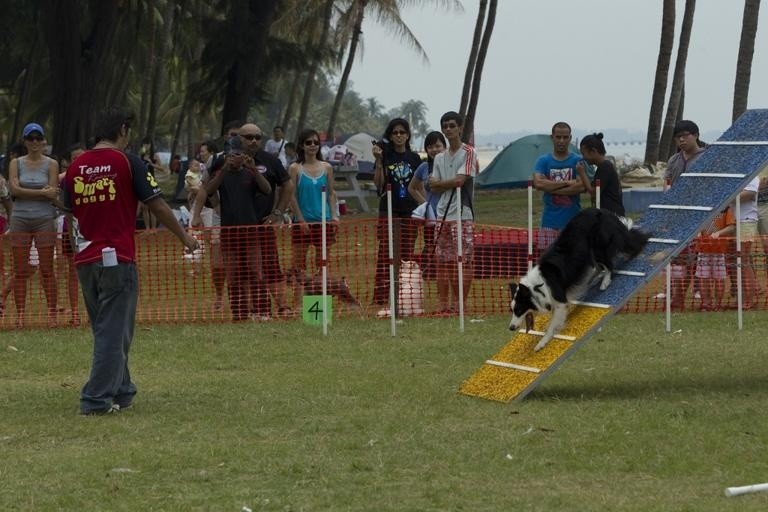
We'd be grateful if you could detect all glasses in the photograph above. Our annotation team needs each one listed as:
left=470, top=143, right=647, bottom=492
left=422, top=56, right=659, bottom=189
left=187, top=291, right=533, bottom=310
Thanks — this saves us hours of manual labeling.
left=441, top=123, right=456, bottom=129
left=392, top=130, right=406, bottom=135
left=244, top=134, right=263, bottom=141
left=26, top=135, right=44, bottom=142
left=305, top=140, right=320, bottom=146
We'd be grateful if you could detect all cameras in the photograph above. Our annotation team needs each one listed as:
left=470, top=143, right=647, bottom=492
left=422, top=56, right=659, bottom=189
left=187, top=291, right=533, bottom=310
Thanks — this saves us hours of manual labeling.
left=233, top=155, right=245, bottom=169
left=372, top=139, right=395, bottom=158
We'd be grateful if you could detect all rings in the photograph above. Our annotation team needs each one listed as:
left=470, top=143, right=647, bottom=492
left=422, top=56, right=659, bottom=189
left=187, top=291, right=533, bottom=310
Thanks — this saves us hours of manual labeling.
left=195, top=243, right=200, bottom=248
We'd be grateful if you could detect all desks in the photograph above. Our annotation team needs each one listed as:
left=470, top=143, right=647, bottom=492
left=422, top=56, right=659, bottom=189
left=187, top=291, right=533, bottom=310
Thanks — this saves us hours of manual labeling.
left=329, top=163, right=372, bottom=214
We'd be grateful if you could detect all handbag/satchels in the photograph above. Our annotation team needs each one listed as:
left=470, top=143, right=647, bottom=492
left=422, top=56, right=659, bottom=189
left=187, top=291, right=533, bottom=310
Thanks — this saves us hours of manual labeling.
left=170, top=155, right=182, bottom=173
left=413, top=224, right=426, bottom=257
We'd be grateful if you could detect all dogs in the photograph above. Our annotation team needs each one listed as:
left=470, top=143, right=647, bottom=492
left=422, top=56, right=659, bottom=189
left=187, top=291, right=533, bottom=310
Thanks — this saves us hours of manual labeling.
left=375, top=259, right=425, bottom=318
left=285, top=268, right=364, bottom=313
left=508, top=206, right=653, bottom=352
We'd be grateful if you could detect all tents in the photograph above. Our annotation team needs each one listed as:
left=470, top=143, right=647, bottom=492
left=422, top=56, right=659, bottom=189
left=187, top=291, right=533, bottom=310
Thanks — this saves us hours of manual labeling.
left=473, top=131, right=583, bottom=188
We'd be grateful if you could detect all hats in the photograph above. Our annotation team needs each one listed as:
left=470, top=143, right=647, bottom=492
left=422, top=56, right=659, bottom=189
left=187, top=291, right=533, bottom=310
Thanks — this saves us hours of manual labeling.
left=22, top=123, right=45, bottom=140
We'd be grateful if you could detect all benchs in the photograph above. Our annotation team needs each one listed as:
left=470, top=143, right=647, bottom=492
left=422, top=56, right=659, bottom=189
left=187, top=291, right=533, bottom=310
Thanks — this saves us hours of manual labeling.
left=359, top=183, right=379, bottom=198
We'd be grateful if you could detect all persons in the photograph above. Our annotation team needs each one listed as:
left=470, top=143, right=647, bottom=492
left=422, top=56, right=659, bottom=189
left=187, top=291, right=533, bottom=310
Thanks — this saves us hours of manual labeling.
left=181, top=121, right=340, bottom=323
left=0, top=123, right=86, bottom=324
left=654, top=120, right=707, bottom=300
left=138, top=137, right=164, bottom=230
left=171, top=155, right=181, bottom=176
left=531, top=122, right=588, bottom=262
left=60, top=103, right=202, bottom=418
left=670, top=177, right=768, bottom=312
left=574, top=131, right=625, bottom=215
left=369, top=111, right=477, bottom=320
